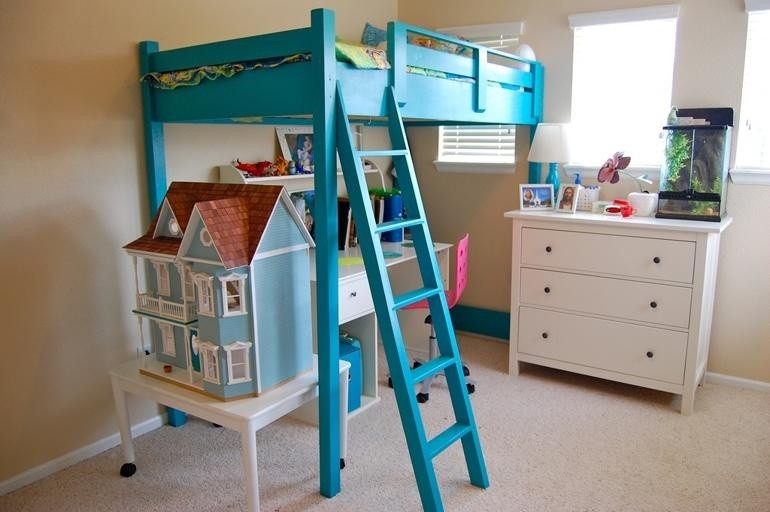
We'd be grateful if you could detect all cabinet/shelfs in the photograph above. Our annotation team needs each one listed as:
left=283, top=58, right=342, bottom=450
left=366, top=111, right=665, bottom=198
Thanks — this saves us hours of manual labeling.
left=504, top=206, right=733, bottom=416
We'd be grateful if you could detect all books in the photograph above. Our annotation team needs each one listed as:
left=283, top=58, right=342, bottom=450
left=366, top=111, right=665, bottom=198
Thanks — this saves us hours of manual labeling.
left=338, top=193, right=384, bottom=251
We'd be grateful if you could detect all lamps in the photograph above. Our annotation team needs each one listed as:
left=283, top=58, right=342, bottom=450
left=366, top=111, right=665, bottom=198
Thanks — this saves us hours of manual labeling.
left=527, top=123, right=582, bottom=204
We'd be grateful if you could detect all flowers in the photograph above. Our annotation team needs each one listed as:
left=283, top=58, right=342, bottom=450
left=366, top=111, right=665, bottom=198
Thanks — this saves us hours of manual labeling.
left=597, top=151, right=659, bottom=192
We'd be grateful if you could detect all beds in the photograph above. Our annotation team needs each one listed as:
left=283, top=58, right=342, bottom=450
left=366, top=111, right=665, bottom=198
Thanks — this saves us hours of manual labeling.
left=138, top=9, right=548, bottom=499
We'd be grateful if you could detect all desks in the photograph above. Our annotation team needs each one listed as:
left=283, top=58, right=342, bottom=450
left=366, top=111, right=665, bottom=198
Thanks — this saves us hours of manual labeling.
left=288, top=240, right=454, bottom=427
left=109, top=353, right=352, bottom=512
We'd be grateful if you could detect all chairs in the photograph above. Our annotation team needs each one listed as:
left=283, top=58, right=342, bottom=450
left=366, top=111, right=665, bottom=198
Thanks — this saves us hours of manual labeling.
left=387, top=232, right=475, bottom=402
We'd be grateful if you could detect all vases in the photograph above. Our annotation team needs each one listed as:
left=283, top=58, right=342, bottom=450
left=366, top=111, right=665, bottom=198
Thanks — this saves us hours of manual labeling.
left=627, top=192, right=658, bottom=217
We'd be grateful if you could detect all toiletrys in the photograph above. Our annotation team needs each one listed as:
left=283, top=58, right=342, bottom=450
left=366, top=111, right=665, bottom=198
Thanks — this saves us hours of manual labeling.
left=288, top=161, right=297, bottom=175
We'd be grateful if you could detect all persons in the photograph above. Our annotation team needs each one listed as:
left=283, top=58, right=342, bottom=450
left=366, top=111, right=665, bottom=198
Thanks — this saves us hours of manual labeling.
left=559, top=187, right=575, bottom=210
left=523, top=189, right=536, bottom=208
left=291, top=135, right=313, bottom=161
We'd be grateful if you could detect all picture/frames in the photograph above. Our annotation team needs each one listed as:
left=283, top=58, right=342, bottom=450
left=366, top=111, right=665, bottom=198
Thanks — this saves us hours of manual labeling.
left=519, top=184, right=554, bottom=211
left=275, top=127, right=313, bottom=172
left=556, top=183, right=581, bottom=214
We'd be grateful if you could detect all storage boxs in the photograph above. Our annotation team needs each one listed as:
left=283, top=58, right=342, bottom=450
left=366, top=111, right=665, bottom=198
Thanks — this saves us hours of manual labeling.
left=340, top=342, right=362, bottom=412
